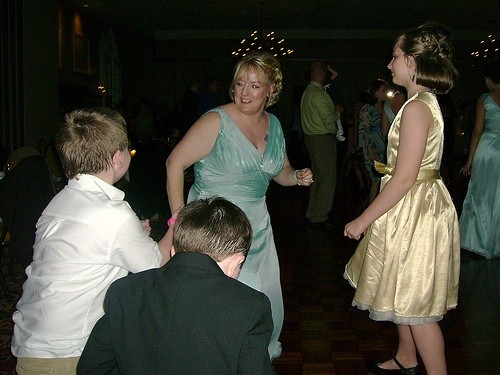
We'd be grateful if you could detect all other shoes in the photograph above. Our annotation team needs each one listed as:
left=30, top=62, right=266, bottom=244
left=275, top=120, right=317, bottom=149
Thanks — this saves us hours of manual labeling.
left=358, top=184, right=365, bottom=191
left=305, top=218, right=334, bottom=231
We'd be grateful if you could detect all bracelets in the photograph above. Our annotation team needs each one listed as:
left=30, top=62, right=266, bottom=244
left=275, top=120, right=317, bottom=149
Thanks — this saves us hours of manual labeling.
left=294, top=170, right=300, bottom=186
left=167, top=218, right=177, bottom=226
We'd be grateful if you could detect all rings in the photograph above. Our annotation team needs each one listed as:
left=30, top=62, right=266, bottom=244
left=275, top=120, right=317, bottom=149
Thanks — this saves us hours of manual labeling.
left=311, top=179, right=314, bottom=183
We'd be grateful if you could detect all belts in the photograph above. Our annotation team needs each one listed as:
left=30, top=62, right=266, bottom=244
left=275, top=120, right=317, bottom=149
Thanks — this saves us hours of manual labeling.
left=374, top=159, right=442, bottom=181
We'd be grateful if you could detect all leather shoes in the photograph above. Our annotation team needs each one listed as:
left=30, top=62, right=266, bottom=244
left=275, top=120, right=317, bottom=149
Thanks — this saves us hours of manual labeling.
left=368, top=356, right=421, bottom=375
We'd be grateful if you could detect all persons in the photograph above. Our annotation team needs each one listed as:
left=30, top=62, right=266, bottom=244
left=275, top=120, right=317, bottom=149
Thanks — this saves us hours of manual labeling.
left=459, top=67, right=500, bottom=260
left=114, top=140, right=160, bottom=200
left=344, top=22, right=460, bottom=375
left=76, top=195, right=277, bottom=375
left=11, top=107, right=185, bottom=375
left=166, top=51, right=314, bottom=361
left=340, top=79, right=408, bottom=206
left=183, top=80, right=200, bottom=130
left=322, top=65, right=346, bottom=141
left=197, top=80, right=227, bottom=120
left=2, top=141, right=68, bottom=248
left=300, top=62, right=343, bottom=227
